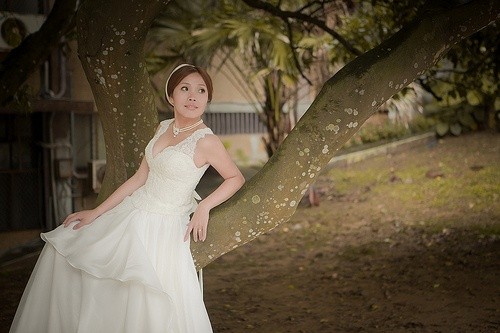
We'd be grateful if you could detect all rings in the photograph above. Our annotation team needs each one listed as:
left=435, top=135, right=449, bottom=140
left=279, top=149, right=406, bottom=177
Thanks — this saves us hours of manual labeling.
left=199, top=229, right=203, bottom=232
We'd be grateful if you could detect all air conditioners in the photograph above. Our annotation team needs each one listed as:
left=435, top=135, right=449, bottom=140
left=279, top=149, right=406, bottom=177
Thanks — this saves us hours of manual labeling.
left=0, top=11, right=49, bottom=51
left=92, top=159, right=107, bottom=193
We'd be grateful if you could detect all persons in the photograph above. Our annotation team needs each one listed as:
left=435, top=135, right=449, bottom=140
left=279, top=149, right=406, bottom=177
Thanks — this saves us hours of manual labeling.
left=7, top=63, right=246, bottom=333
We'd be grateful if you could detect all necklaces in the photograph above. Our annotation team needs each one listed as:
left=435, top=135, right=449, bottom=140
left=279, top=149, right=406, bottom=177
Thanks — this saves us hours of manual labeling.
left=173, top=119, right=203, bottom=137
